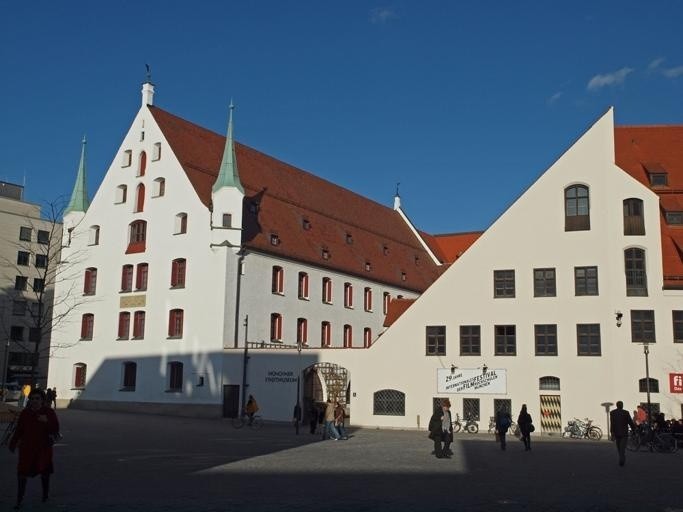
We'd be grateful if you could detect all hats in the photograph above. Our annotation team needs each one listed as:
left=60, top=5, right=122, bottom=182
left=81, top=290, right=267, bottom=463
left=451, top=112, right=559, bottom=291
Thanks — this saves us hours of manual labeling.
left=441, top=400, right=451, bottom=407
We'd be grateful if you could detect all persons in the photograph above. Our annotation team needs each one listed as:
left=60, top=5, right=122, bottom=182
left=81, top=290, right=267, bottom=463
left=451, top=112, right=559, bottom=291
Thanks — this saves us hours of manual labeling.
left=610, top=401, right=636, bottom=466
left=18, top=382, right=57, bottom=409
left=242, top=394, right=259, bottom=426
left=495, top=403, right=512, bottom=450
left=9, top=388, right=59, bottom=510
left=635, top=406, right=682, bottom=435
left=309, top=398, right=348, bottom=440
left=517, top=403, right=535, bottom=451
left=430, top=399, right=454, bottom=458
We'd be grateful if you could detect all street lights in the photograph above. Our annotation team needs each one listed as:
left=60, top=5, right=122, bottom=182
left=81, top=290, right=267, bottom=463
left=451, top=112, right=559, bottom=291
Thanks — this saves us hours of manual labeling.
left=2, top=336, right=14, bottom=402
left=638, top=341, right=654, bottom=424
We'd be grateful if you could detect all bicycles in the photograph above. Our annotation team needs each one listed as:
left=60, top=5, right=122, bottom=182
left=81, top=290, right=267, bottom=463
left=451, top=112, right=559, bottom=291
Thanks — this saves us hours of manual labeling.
left=231, top=406, right=263, bottom=429
left=626, top=424, right=679, bottom=453
left=562, top=418, right=604, bottom=439
left=487, top=418, right=518, bottom=440
left=451, top=413, right=479, bottom=433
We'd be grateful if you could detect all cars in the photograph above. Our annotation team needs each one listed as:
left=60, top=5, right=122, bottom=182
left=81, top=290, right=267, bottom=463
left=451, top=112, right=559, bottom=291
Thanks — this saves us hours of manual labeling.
left=2, top=381, right=22, bottom=402
